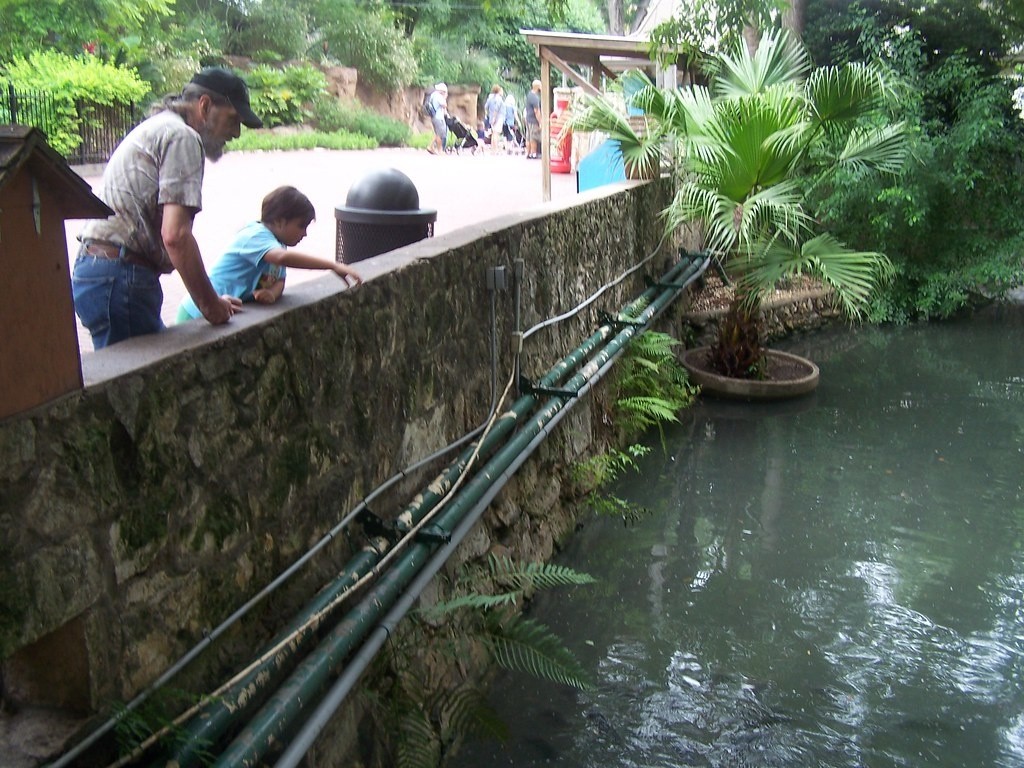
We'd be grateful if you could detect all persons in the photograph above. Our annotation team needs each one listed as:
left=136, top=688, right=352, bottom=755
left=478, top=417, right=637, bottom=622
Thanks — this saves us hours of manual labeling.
left=426, top=82, right=448, bottom=155
left=72, top=66, right=264, bottom=352
left=176, top=185, right=363, bottom=322
left=483, top=84, right=521, bottom=156
left=525, top=79, right=542, bottom=159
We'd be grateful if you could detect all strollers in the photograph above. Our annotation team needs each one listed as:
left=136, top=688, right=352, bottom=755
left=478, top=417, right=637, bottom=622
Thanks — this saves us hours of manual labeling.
left=444, top=114, right=483, bottom=156
left=504, top=122, right=525, bottom=155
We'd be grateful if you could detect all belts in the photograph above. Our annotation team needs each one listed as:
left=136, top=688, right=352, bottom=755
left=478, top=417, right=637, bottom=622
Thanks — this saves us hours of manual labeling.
left=79, top=243, right=161, bottom=278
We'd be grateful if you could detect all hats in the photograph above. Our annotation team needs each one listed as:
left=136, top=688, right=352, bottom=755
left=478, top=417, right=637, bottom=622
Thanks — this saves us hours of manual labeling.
left=189, top=67, right=264, bottom=129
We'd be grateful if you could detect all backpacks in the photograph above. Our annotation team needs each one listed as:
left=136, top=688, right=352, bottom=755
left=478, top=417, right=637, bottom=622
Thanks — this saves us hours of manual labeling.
left=421, top=95, right=440, bottom=117
